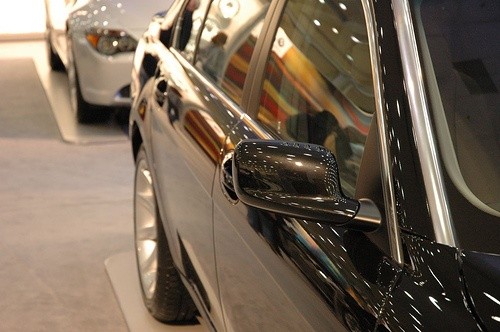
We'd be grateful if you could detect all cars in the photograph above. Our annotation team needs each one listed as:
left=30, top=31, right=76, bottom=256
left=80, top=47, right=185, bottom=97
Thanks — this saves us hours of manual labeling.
left=45, top=1, right=177, bottom=125
left=129, top=0, right=499, bottom=331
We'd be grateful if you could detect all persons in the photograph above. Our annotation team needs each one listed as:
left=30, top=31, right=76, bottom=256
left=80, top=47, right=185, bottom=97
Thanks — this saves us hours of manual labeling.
left=196, top=31, right=228, bottom=87
left=275, top=107, right=353, bottom=173
